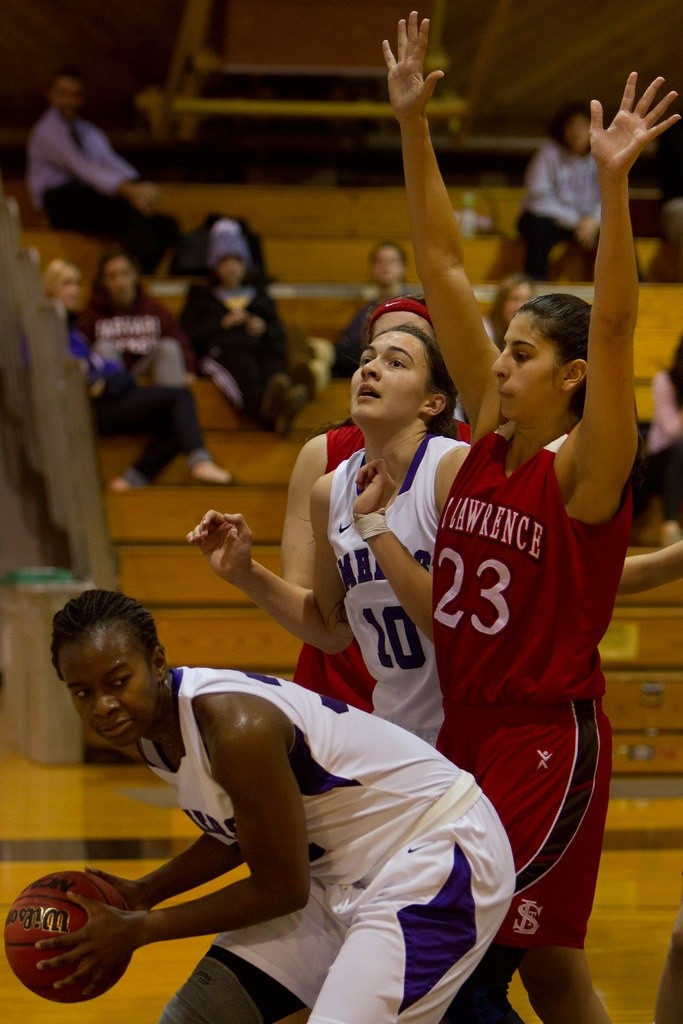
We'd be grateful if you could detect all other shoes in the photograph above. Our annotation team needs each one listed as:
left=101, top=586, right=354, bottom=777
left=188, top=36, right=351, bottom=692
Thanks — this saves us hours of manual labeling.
left=185, top=461, right=245, bottom=488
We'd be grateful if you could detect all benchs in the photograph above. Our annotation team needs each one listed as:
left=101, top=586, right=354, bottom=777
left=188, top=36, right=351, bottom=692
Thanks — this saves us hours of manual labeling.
left=169, top=94, right=468, bottom=145
left=172, top=0, right=452, bottom=93
left=0, top=181, right=683, bottom=777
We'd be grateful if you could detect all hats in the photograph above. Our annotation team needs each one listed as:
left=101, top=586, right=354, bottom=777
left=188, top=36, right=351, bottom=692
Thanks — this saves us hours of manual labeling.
left=208, top=217, right=249, bottom=269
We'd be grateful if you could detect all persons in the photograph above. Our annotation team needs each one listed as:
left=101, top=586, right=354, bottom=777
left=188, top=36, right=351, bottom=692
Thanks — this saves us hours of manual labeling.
left=16, top=260, right=125, bottom=443
left=326, top=239, right=425, bottom=379
left=280, top=292, right=474, bottom=716
left=372, top=9, right=680, bottom=1023
left=188, top=323, right=473, bottom=757
left=76, top=245, right=229, bottom=493
left=482, top=272, right=533, bottom=354
left=174, top=219, right=294, bottom=437
left=643, top=327, right=680, bottom=544
left=38, top=589, right=518, bottom=1022
left=515, top=93, right=646, bottom=285
left=24, top=64, right=183, bottom=277
left=648, top=195, right=681, bottom=281
